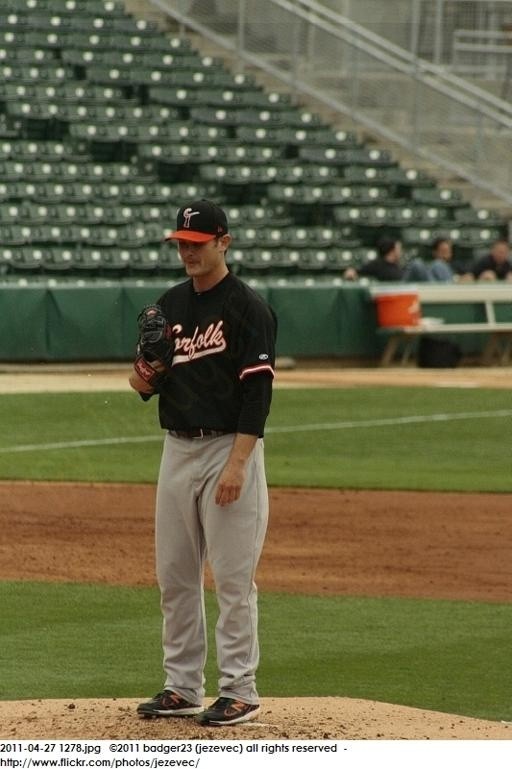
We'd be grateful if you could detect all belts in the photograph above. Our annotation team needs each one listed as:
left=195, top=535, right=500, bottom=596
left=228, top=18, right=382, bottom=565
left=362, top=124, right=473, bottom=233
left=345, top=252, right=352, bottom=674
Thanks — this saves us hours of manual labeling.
left=164, top=424, right=231, bottom=441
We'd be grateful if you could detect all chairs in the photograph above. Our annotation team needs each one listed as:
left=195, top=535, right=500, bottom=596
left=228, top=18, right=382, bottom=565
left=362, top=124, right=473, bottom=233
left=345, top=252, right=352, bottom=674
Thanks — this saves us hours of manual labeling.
left=0, top=0, right=512, bottom=286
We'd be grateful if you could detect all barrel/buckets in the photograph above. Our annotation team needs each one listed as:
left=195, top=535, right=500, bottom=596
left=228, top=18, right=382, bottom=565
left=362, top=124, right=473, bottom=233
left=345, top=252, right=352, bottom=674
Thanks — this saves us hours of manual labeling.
left=373, top=287, right=421, bottom=326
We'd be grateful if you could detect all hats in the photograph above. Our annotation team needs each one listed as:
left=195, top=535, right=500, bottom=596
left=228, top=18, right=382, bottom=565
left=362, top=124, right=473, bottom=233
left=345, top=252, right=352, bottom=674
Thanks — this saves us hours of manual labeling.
left=163, top=198, right=228, bottom=243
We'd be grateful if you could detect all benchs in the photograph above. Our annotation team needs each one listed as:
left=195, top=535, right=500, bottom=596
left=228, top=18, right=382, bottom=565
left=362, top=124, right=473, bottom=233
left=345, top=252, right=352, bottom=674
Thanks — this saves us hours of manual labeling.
left=369, top=287, right=512, bottom=364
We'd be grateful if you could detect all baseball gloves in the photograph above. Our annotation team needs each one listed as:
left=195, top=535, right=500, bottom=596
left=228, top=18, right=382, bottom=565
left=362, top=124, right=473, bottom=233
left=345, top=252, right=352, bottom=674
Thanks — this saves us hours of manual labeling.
left=130, top=304, right=176, bottom=386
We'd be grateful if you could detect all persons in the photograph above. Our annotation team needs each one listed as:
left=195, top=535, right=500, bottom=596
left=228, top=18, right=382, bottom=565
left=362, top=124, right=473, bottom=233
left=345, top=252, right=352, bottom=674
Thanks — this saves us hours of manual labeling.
left=344, top=233, right=451, bottom=285
left=429, top=235, right=477, bottom=283
left=126, top=200, right=278, bottom=729
left=465, top=239, right=512, bottom=283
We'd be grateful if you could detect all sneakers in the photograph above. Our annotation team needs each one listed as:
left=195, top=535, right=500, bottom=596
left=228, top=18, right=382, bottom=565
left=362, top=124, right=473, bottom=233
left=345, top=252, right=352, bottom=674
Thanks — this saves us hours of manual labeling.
left=193, top=691, right=260, bottom=726
left=136, top=687, right=204, bottom=716
